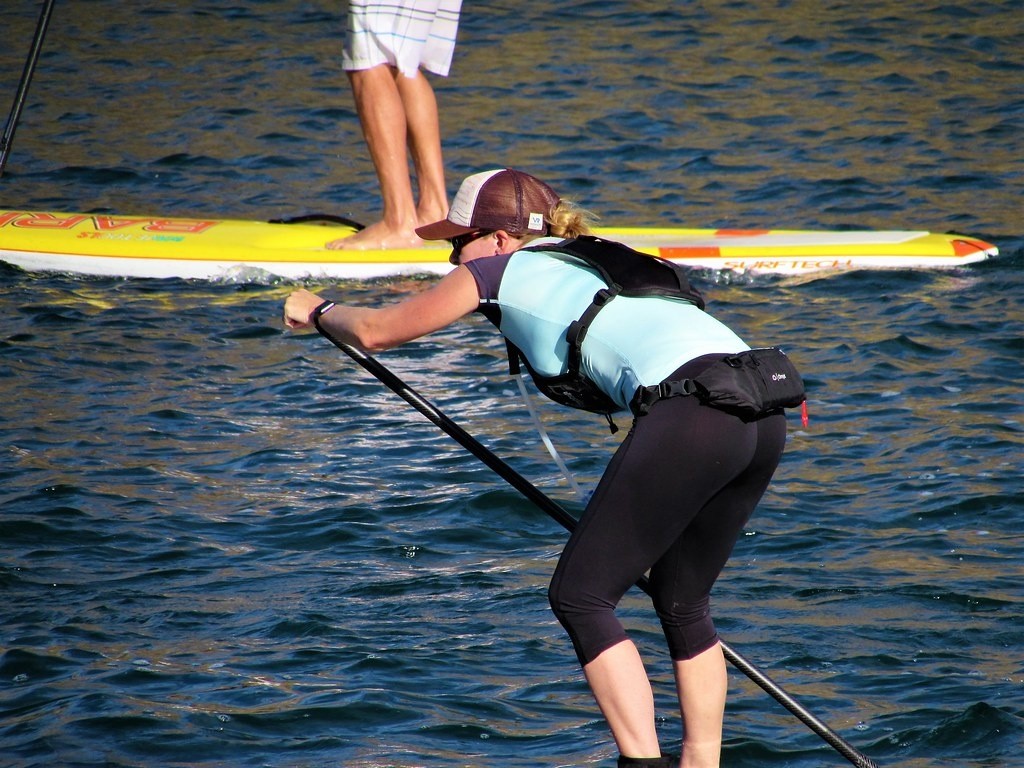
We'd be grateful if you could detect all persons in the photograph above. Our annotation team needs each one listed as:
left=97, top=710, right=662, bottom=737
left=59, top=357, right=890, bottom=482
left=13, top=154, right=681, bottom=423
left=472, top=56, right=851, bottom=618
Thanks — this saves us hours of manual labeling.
left=284, top=168, right=788, bottom=768
left=326, top=0, right=467, bottom=250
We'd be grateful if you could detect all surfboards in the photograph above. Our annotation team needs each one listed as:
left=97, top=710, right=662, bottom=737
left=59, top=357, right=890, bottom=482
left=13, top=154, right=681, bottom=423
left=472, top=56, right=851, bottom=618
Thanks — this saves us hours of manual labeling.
left=0, top=205, right=998, bottom=286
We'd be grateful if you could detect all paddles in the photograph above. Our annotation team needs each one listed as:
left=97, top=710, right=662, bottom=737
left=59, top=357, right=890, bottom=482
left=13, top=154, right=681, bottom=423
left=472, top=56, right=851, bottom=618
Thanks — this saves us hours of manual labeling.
left=0, top=0, right=56, bottom=170
left=309, top=312, right=875, bottom=768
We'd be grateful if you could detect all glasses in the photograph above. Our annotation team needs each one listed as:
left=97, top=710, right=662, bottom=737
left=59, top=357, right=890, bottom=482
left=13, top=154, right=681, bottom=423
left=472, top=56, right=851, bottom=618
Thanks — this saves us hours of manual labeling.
left=452, top=229, right=496, bottom=250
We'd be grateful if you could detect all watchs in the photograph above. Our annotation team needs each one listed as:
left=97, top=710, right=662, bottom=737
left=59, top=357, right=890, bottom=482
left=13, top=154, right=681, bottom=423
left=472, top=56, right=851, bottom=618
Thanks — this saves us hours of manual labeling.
left=314, top=301, right=335, bottom=328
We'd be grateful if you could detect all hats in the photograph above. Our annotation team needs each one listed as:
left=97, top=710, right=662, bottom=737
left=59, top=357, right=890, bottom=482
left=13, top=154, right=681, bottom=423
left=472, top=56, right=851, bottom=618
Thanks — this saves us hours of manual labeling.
left=415, top=167, right=562, bottom=241
